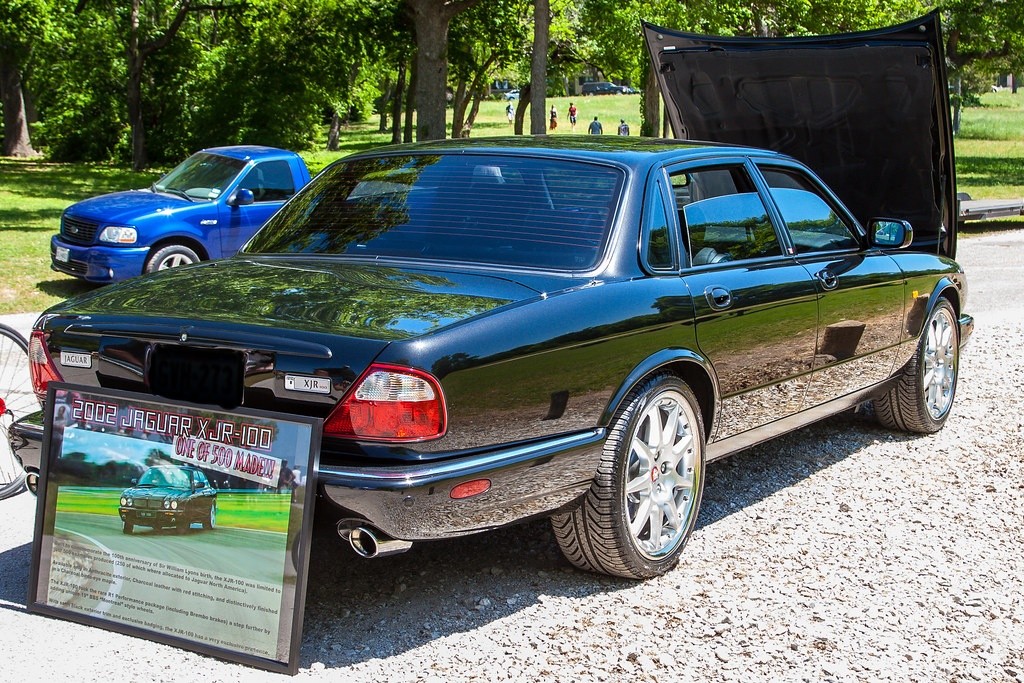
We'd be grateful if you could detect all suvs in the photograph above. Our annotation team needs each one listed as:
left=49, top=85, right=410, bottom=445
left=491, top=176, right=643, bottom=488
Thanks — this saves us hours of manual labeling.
left=116, top=464, right=218, bottom=534
left=581, top=82, right=628, bottom=95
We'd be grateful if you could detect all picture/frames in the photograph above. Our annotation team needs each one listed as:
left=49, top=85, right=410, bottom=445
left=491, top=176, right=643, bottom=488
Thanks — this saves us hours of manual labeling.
left=25, top=380, right=322, bottom=675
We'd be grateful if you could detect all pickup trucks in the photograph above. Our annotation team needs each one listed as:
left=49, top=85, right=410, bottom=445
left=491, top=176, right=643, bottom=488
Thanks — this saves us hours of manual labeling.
left=47, top=143, right=441, bottom=284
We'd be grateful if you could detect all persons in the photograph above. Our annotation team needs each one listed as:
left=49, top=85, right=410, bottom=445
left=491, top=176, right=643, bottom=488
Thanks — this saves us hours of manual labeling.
left=550, top=104, right=558, bottom=130
left=588, top=116, right=603, bottom=134
left=506, top=101, right=512, bottom=124
left=617, top=119, right=629, bottom=136
left=462, top=119, right=471, bottom=137
left=567, top=102, right=577, bottom=131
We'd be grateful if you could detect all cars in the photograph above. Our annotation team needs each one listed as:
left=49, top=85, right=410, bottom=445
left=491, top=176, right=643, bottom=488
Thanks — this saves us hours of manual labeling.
left=503, top=89, right=520, bottom=100
left=4, top=8, right=978, bottom=581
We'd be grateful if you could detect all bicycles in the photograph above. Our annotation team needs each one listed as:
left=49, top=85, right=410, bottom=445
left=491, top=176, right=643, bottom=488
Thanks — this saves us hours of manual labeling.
left=0, top=324, right=44, bottom=499
